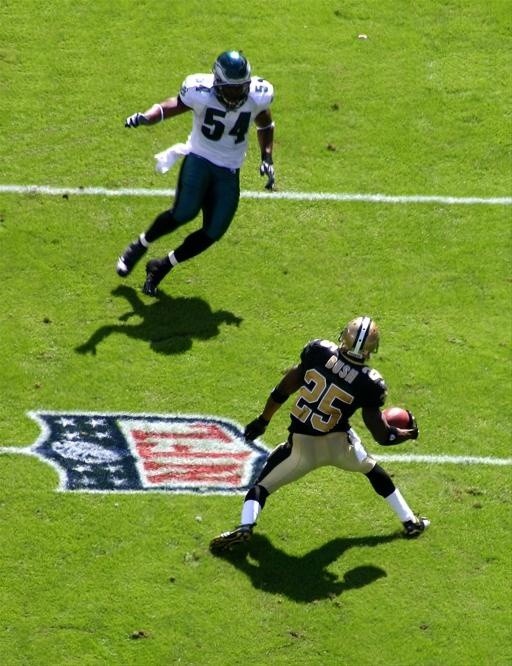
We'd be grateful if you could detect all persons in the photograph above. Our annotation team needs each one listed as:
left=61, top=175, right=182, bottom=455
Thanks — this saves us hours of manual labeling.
left=114, top=49, right=277, bottom=296
left=210, top=315, right=430, bottom=558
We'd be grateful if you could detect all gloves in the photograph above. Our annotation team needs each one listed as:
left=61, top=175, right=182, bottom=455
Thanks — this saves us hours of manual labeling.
left=405, top=410, right=420, bottom=443
left=124, top=111, right=148, bottom=129
left=259, top=159, right=275, bottom=192
left=240, top=415, right=271, bottom=445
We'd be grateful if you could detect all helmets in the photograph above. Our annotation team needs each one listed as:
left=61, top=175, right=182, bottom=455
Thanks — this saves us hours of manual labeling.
left=336, top=317, right=382, bottom=359
left=212, top=49, right=253, bottom=93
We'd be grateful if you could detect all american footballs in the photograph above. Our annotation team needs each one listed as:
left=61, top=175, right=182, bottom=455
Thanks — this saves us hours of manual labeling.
left=380, top=407, right=409, bottom=430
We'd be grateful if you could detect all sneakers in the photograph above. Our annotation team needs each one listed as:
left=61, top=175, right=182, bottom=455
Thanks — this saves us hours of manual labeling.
left=402, top=513, right=433, bottom=540
left=142, top=260, right=165, bottom=298
left=114, top=242, right=142, bottom=277
left=209, top=523, right=254, bottom=557
